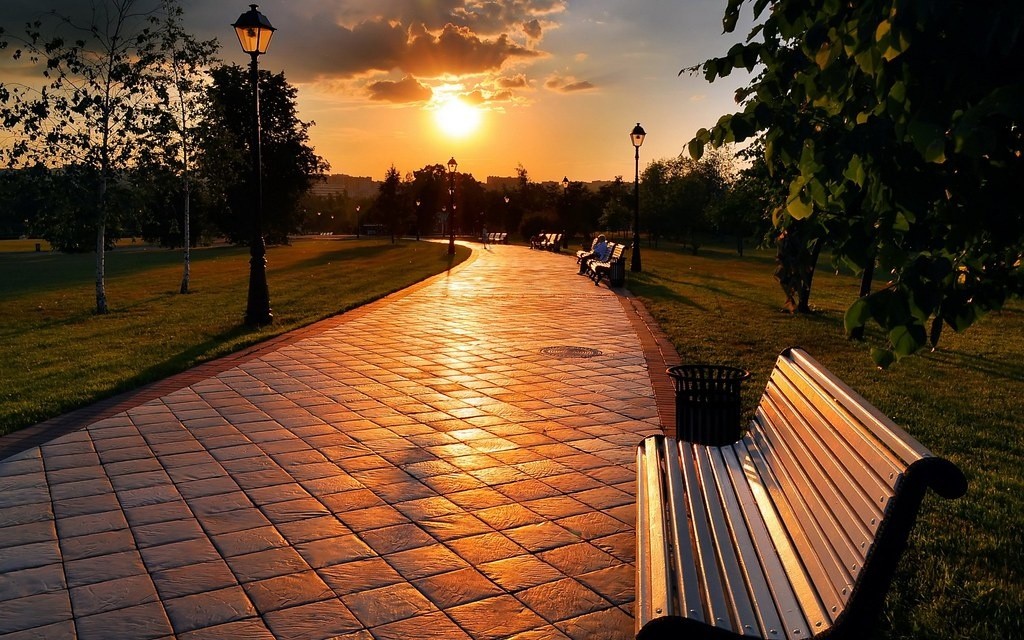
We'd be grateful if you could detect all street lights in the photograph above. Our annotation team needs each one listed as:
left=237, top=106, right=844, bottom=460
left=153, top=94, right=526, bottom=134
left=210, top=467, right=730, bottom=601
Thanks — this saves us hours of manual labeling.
left=356, top=206, right=360, bottom=237
left=451, top=201, right=457, bottom=236
left=442, top=204, right=448, bottom=237
left=479, top=211, right=484, bottom=236
left=229, top=3, right=278, bottom=327
left=630, top=122, right=647, bottom=273
left=447, top=156, right=458, bottom=255
left=562, top=175, right=570, bottom=249
left=415, top=199, right=421, bottom=240
left=503, top=194, right=510, bottom=244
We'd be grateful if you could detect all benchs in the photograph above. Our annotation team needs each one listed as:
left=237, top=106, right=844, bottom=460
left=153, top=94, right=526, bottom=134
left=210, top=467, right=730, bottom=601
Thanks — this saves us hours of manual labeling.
left=576, top=238, right=628, bottom=286
left=633, top=346, right=970, bottom=640
left=479, top=232, right=507, bottom=245
left=532, top=233, right=562, bottom=249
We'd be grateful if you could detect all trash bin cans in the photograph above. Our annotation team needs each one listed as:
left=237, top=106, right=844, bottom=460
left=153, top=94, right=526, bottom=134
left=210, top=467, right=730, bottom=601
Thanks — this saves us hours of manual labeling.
left=665, top=363, right=750, bottom=446
left=503, top=237, right=508, bottom=244
left=554, top=240, right=560, bottom=253
left=608, top=256, right=627, bottom=288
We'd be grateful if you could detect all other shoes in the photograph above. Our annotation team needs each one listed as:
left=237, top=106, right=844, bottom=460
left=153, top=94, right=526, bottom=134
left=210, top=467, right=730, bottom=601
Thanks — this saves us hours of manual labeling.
left=577, top=272, right=584, bottom=275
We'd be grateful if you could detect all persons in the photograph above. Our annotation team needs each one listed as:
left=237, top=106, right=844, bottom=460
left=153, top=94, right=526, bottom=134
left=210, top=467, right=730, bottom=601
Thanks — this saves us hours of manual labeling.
left=482, top=224, right=488, bottom=249
left=577, top=235, right=606, bottom=275
left=528, top=230, right=545, bottom=250
